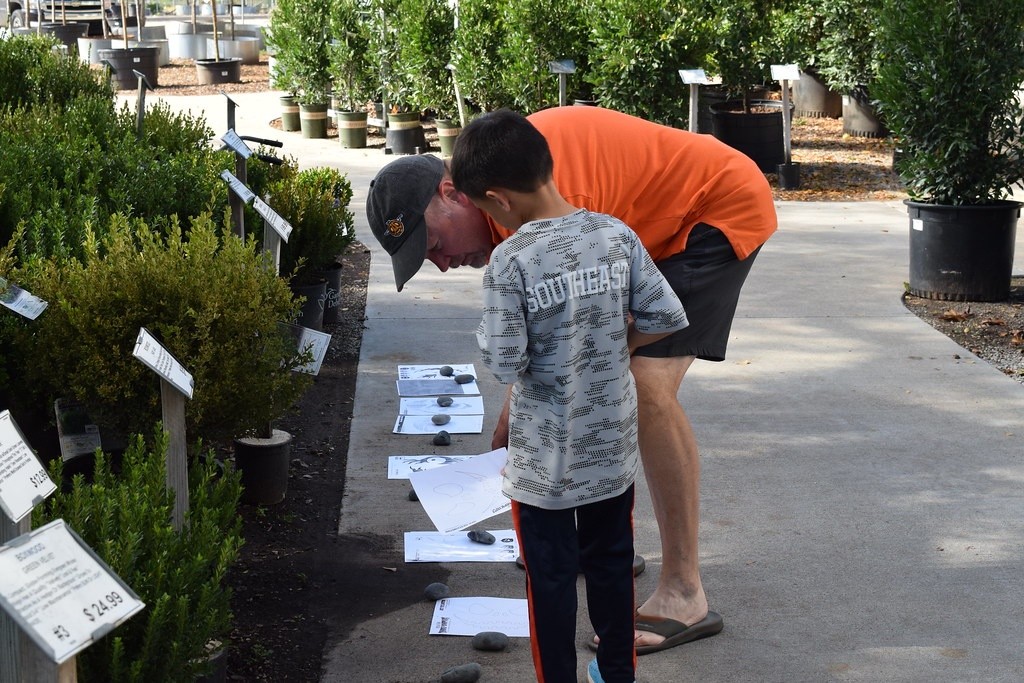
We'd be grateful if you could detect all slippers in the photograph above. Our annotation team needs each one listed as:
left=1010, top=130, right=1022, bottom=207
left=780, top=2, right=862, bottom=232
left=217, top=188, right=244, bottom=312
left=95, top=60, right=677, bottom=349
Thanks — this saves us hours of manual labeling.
left=587, top=603, right=724, bottom=655
left=515, top=554, right=646, bottom=577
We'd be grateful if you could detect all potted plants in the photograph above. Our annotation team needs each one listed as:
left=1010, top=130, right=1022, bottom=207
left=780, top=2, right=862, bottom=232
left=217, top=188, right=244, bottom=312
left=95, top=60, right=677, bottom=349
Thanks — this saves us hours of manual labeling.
left=0, top=0, right=1024, bottom=683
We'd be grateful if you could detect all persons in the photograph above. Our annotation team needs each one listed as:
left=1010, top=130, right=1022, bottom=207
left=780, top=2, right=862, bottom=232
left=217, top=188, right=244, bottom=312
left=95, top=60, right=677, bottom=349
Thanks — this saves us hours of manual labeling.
left=367, top=106, right=779, bottom=656
left=451, top=111, right=691, bottom=683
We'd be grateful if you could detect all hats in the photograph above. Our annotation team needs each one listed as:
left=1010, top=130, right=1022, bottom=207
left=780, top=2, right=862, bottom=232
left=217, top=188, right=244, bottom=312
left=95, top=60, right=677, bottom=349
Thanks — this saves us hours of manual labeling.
left=366, top=153, right=444, bottom=292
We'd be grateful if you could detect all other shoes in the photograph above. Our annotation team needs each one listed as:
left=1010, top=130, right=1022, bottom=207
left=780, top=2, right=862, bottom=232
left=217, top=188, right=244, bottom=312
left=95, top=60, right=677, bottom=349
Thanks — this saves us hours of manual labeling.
left=588, top=659, right=605, bottom=683
